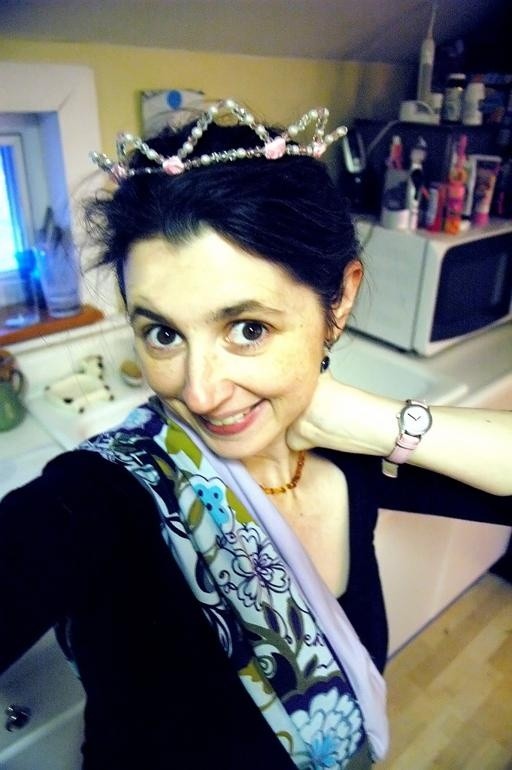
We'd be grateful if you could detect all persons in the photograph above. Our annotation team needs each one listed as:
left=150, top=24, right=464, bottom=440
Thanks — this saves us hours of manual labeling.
left=0, top=117, right=511, bottom=769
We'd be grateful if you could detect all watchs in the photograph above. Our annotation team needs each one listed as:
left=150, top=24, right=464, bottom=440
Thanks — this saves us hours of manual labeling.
left=381, top=399, right=433, bottom=478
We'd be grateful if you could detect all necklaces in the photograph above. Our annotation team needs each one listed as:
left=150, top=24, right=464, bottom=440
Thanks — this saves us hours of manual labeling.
left=258, top=450, right=306, bottom=494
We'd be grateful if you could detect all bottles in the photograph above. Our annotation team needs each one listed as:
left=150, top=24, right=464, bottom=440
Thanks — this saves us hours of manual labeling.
left=443, top=70, right=466, bottom=123
left=463, top=74, right=485, bottom=127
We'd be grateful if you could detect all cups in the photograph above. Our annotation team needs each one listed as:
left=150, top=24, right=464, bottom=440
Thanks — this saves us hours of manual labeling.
left=1, top=273, right=40, bottom=329
left=0, top=348, right=29, bottom=433
left=33, top=239, right=82, bottom=319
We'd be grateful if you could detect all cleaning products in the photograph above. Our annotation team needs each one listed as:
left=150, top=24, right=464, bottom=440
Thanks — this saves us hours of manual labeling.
left=32, top=206, right=82, bottom=317
